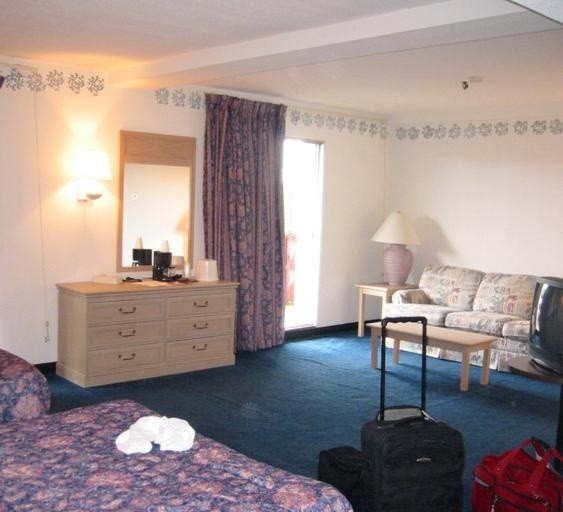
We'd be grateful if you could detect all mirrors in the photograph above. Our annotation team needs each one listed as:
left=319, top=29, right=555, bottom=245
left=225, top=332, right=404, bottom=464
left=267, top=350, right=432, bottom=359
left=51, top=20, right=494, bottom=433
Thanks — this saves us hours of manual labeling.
left=115, top=129, right=197, bottom=272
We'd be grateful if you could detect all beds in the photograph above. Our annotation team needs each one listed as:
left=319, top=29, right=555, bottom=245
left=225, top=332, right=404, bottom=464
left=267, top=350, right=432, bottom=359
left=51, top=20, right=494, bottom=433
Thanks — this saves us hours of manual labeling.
left=0, top=398, right=353, bottom=512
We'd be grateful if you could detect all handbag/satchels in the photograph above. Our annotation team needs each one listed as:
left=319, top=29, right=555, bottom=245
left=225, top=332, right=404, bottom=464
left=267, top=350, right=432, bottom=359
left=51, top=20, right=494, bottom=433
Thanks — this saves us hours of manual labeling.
left=317, top=446, right=362, bottom=512
left=470, top=436, right=563, bottom=512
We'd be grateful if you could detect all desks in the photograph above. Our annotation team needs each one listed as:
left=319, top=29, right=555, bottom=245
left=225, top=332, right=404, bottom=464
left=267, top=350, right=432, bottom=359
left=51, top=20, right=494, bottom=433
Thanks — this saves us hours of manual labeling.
left=365, top=321, right=498, bottom=392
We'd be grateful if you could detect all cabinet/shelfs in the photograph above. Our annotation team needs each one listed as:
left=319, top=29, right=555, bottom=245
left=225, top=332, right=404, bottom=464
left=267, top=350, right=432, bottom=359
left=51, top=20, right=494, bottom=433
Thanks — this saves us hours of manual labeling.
left=57, top=282, right=240, bottom=389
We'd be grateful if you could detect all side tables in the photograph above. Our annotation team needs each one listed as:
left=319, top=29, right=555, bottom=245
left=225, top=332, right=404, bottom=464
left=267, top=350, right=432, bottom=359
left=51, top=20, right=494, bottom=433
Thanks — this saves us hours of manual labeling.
left=357, top=284, right=418, bottom=337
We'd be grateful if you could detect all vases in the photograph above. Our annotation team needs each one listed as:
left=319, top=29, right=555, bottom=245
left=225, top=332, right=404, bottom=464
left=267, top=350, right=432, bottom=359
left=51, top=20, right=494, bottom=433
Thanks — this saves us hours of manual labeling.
left=383, top=245, right=413, bottom=286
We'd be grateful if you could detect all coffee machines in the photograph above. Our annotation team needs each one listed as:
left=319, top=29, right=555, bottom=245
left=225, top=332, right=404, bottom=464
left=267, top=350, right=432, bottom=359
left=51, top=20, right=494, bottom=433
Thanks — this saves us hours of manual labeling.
left=132, top=249, right=152, bottom=268
left=151, top=252, right=177, bottom=283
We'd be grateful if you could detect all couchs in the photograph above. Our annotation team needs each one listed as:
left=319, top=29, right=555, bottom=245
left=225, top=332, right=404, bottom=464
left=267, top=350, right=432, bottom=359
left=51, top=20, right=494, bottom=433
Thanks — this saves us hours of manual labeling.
left=382, top=265, right=545, bottom=372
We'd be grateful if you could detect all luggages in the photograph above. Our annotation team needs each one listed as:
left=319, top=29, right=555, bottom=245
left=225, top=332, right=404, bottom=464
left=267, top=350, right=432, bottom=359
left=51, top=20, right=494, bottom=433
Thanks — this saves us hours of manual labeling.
left=360, top=316, right=466, bottom=511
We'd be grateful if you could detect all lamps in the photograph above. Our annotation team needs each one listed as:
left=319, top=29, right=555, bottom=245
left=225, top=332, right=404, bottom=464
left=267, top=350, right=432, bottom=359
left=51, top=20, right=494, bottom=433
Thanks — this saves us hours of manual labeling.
left=370, top=211, right=421, bottom=246
left=78, top=147, right=113, bottom=201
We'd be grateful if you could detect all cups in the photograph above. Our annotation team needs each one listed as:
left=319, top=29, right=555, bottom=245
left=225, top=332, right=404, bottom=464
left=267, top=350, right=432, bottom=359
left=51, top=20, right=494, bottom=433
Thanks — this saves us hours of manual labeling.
left=183, top=262, right=193, bottom=280
left=162, top=240, right=169, bottom=254
left=136, top=238, right=143, bottom=250
